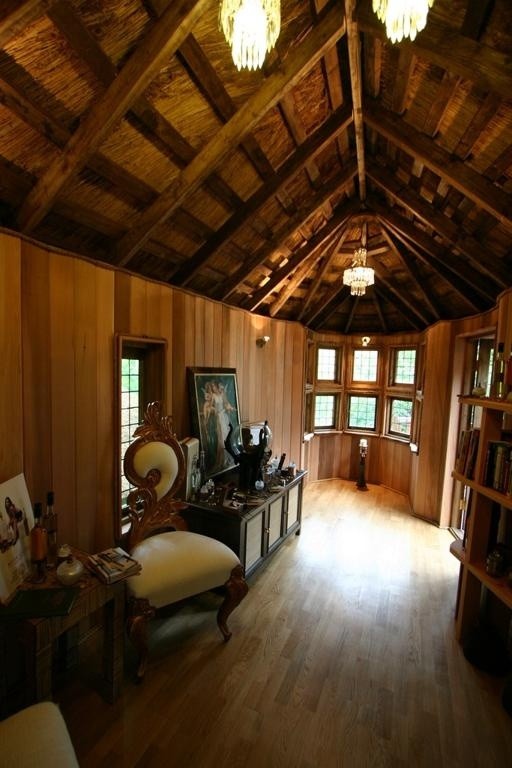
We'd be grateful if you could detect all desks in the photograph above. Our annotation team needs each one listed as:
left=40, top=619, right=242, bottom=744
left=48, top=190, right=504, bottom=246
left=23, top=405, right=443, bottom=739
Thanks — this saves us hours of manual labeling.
left=0, top=537, right=121, bottom=705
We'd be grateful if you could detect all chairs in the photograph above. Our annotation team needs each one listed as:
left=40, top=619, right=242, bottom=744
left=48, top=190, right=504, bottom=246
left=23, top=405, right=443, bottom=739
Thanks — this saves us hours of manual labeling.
left=0, top=702, right=81, bottom=768
left=115, top=400, right=249, bottom=685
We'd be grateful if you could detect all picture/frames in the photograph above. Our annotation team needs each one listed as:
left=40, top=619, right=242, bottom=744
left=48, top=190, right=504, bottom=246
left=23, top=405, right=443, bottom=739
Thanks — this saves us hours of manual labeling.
left=185, top=365, right=242, bottom=480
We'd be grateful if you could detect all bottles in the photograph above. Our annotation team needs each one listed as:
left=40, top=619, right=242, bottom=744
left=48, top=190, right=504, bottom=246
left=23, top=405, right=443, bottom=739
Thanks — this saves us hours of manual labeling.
left=41, top=492, right=59, bottom=546
left=505, top=343, right=512, bottom=395
left=31, top=501, right=49, bottom=562
left=191, top=450, right=216, bottom=504
left=254, top=456, right=296, bottom=500
left=57, top=553, right=84, bottom=588
left=491, top=341, right=505, bottom=400
left=483, top=549, right=506, bottom=578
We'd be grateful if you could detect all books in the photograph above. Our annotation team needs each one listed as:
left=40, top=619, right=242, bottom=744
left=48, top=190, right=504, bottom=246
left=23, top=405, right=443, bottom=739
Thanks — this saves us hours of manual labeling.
left=453, top=429, right=512, bottom=498
left=4, top=586, right=80, bottom=618
left=84, top=547, right=142, bottom=584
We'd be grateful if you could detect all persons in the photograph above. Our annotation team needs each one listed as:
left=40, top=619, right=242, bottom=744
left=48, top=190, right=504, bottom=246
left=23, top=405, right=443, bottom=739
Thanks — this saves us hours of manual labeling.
left=203, top=380, right=236, bottom=473
left=5, top=496, right=23, bottom=544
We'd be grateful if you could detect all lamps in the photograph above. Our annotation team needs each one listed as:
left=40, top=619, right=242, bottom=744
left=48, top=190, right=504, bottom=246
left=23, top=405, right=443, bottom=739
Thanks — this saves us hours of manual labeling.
left=216, top=0, right=281, bottom=73
left=372, top=0, right=435, bottom=44
left=256, top=336, right=270, bottom=347
left=342, top=227, right=374, bottom=296
left=361, top=336, right=370, bottom=347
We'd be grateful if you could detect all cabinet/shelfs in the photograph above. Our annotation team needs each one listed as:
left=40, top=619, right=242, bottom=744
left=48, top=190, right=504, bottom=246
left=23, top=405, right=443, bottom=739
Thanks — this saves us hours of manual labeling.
left=185, top=469, right=308, bottom=580
left=449, top=394, right=512, bottom=641
